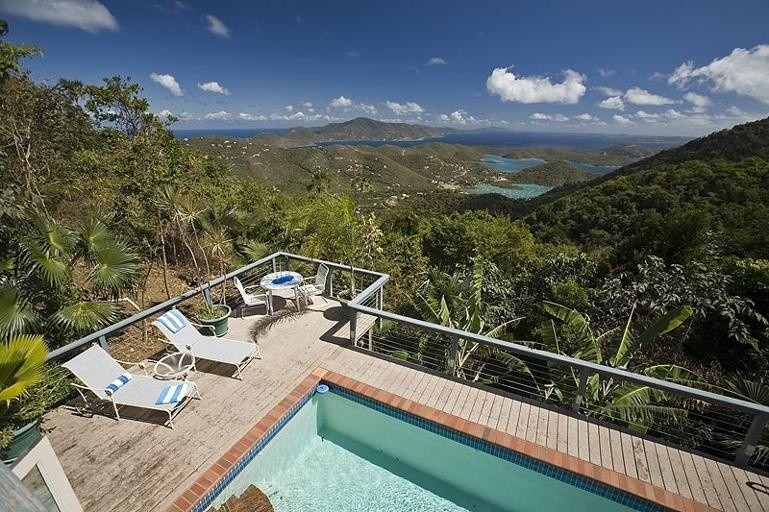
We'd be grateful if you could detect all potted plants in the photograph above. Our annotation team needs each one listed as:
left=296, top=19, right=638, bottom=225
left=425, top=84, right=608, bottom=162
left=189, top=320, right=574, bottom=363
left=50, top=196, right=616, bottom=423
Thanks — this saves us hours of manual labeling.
left=319, top=190, right=369, bottom=322
left=154, top=183, right=234, bottom=338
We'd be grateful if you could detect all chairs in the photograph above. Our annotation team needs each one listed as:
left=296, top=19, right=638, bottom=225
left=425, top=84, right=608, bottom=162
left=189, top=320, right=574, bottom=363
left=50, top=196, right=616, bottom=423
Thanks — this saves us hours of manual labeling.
left=233, top=275, right=269, bottom=318
left=58, top=341, right=203, bottom=430
left=149, top=308, right=262, bottom=382
left=300, top=261, right=329, bottom=311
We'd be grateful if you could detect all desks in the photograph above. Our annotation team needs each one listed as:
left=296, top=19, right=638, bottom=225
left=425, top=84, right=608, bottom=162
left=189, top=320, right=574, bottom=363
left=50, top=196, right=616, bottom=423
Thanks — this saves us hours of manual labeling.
left=261, top=271, right=302, bottom=316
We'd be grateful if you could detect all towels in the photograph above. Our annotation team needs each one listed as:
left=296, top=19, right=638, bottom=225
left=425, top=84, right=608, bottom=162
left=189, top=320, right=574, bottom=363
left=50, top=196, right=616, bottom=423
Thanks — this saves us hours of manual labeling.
left=106, top=369, right=132, bottom=395
left=159, top=310, right=191, bottom=334
left=155, top=385, right=187, bottom=405
